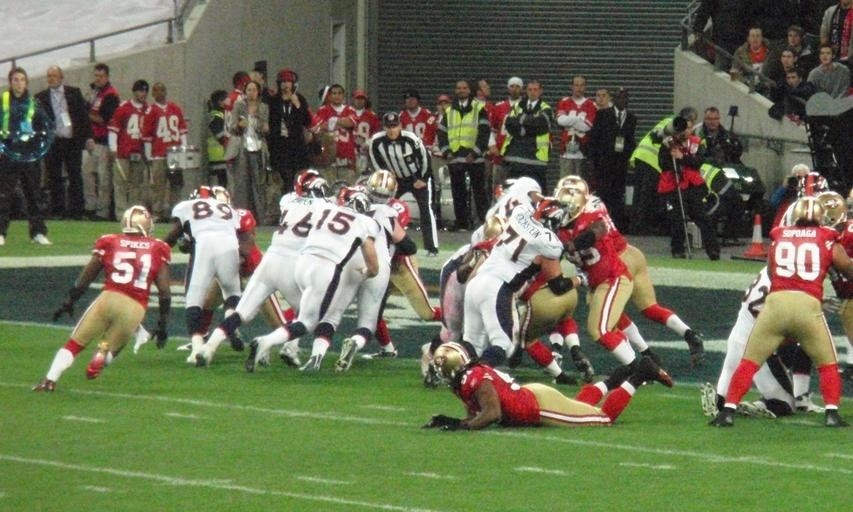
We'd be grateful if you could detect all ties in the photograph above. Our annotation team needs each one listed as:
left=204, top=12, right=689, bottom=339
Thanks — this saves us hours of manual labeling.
left=617, top=112, right=622, bottom=128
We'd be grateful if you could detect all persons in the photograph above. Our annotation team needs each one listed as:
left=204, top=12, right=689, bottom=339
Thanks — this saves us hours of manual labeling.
left=141, top=83, right=188, bottom=222
left=81, top=63, right=119, bottom=221
left=106, top=80, right=156, bottom=222
left=35, top=66, right=90, bottom=219
left=696, top=1, right=852, bottom=200
left=33, top=206, right=172, bottom=393
left=700, top=171, right=852, bottom=434
left=1, top=67, right=53, bottom=245
left=419, top=176, right=705, bottom=433
left=207, top=68, right=827, bottom=260
left=164, top=166, right=442, bottom=377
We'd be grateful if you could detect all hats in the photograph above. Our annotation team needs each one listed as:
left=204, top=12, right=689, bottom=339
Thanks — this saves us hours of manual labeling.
left=383, top=111, right=400, bottom=127
left=508, top=77, right=523, bottom=89
left=403, top=89, right=419, bottom=100
left=438, top=94, right=449, bottom=103
left=353, top=89, right=366, bottom=100
left=276, top=69, right=294, bottom=83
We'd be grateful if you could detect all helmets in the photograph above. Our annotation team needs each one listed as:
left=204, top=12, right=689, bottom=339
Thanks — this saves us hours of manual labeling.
left=433, top=341, right=469, bottom=382
left=483, top=216, right=509, bottom=242
left=533, top=175, right=589, bottom=229
left=792, top=172, right=853, bottom=226
left=121, top=205, right=152, bottom=237
left=294, top=169, right=398, bottom=214
left=189, top=185, right=231, bottom=205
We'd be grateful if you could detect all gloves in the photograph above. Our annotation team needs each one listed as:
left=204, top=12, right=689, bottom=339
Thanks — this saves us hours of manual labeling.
left=53, top=293, right=76, bottom=323
left=151, top=320, right=169, bottom=350
left=577, top=271, right=590, bottom=288
left=565, top=249, right=585, bottom=268
left=821, top=295, right=842, bottom=315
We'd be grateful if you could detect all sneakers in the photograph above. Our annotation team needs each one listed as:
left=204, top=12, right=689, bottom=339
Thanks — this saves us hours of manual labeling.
left=32, top=376, right=57, bottom=392
left=175, top=327, right=396, bottom=374
left=447, top=218, right=473, bottom=232
left=699, top=380, right=852, bottom=427
left=550, top=331, right=704, bottom=388
left=86, top=339, right=110, bottom=379
left=1, top=235, right=6, bottom=245
left=428, top=248, right=436, bottom=258
left=671, top=241, right=685, bottom=258
left=702, top=241, right=721, bottom=259
left=421, top=341, right=441, bottom=388
left=31, top=234, right=53, bottom=245
left=47, top=213, right=119, bottom=222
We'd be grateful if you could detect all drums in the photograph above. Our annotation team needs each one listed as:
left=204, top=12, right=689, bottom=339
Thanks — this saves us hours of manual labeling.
left=166, top=146, right=202, bottom=174
